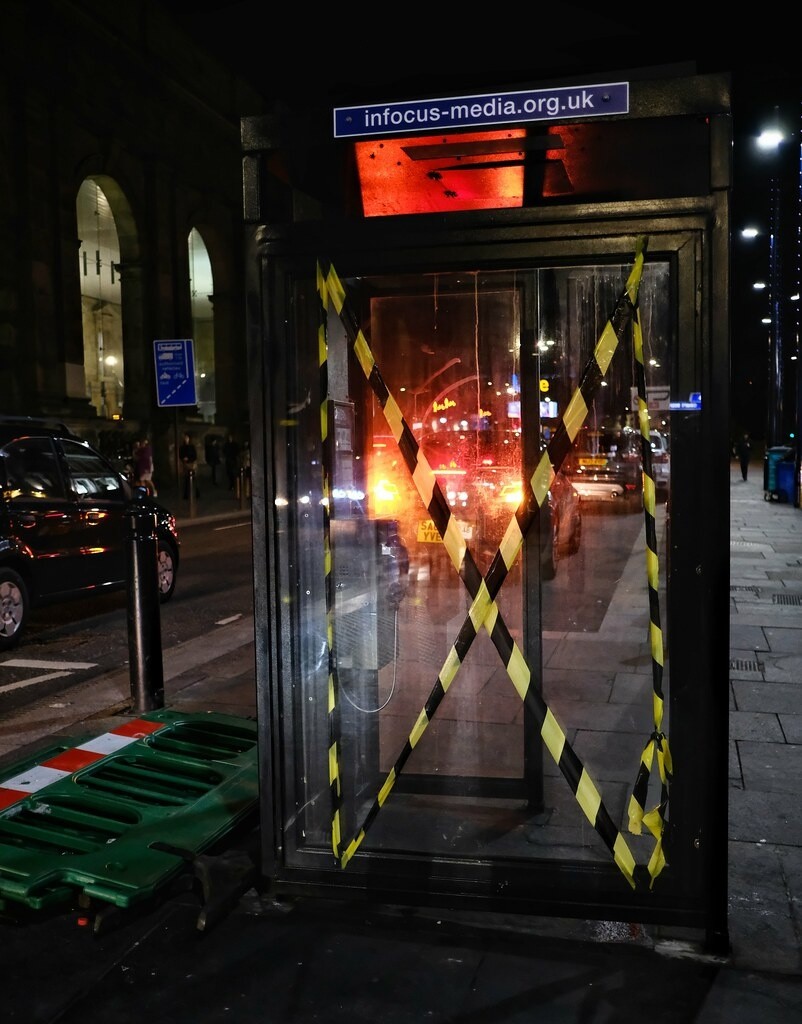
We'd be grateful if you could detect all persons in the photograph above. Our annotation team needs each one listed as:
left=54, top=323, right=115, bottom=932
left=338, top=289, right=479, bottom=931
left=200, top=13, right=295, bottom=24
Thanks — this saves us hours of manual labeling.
left=734, top=434, right=753, bottom=481
left=91, top=426, right=253, bottom=497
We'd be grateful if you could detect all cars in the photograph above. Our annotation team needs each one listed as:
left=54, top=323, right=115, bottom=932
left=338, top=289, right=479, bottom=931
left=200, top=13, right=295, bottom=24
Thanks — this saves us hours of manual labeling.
left=635, top=428, right=668, bottom=502
left=447, top=464, right=582, bottom=580
left=565, top=429, right=644, bottom=512
left=0, top=426, right=181, bottom=650
